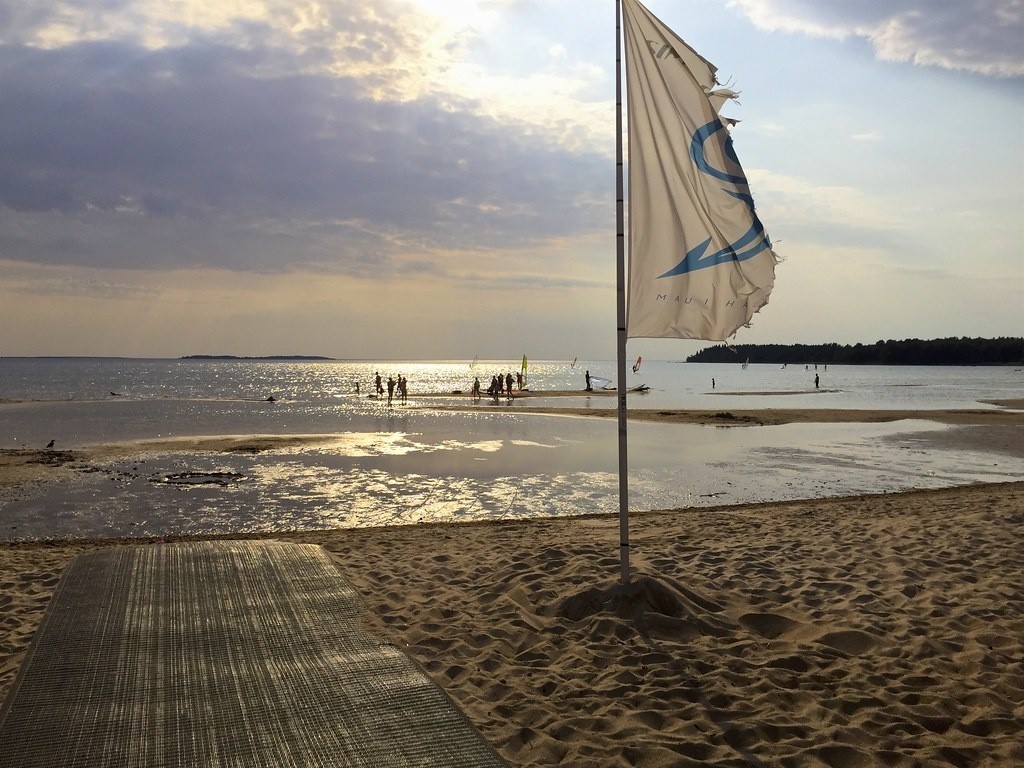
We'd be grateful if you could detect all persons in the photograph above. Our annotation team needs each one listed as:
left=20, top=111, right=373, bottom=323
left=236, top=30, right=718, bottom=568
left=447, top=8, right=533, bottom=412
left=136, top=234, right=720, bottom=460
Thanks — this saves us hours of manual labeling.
left=585, top=371, right=591, bottom=390
left=825, top=364, right=827, bottom=370
left=356, top=372, right=408, bottom=405
left=712, top=378, right=715, bottom=388
left=814, top=374, right=819, bottom=388
left=474, top=372, right=521, bottom=402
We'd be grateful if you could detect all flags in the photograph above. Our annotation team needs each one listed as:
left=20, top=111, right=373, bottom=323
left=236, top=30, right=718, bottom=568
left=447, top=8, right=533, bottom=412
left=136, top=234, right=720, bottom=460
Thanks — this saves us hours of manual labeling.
left=621, top=0, right=776, bottom=342
